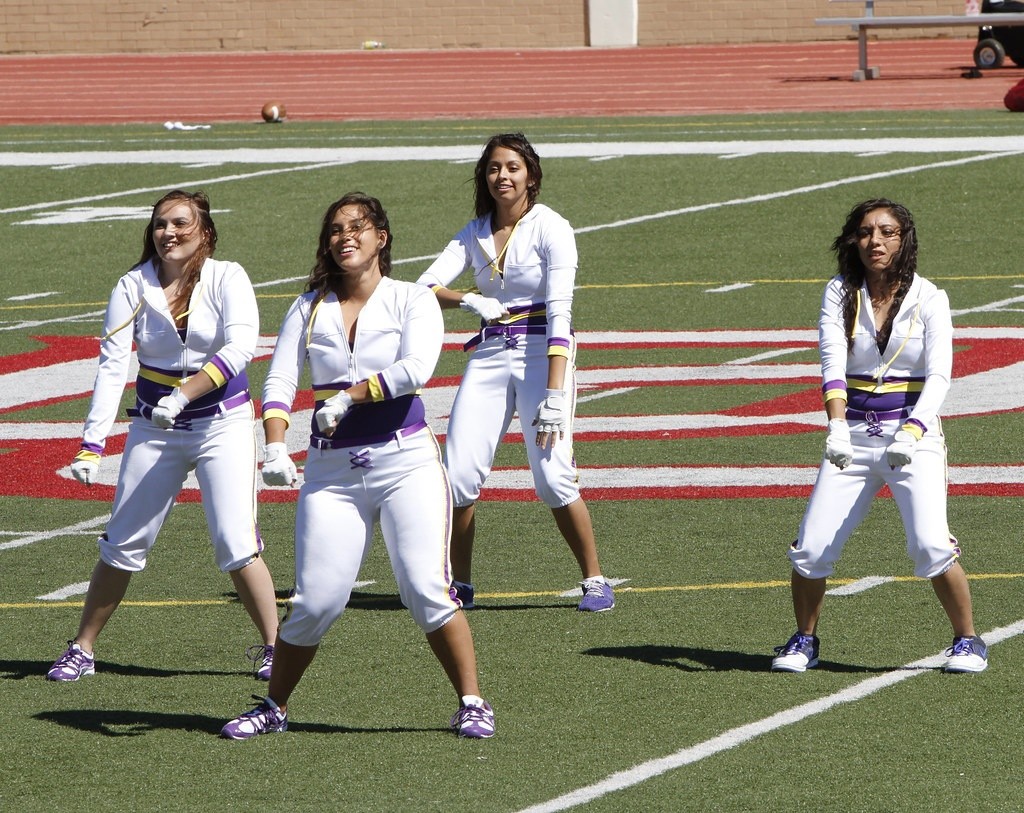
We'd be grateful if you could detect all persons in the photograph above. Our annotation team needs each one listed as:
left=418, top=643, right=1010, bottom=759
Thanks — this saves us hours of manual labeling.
left=772, top=199, right=990, bottom=673
left=413, top=133, right=616, bottom=613
left=221, top=191, right=496, bottom=740
left=45, top=190, right=281, bottom=681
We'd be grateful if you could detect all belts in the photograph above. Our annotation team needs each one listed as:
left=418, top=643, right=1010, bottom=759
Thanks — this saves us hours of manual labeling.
left=311, top=419, right=427, bottom=450
left=463, top=326, right=574, bottom=353
left=135, top=391, right=251, bottom=420
left=846, top=409, right=915, bottom=422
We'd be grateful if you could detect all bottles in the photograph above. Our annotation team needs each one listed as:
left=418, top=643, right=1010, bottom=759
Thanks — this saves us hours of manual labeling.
left=361, top=41, right=386, bottom=49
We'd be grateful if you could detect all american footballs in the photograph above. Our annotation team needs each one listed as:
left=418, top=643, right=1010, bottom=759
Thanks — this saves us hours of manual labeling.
left=262, top=101, right=286, bottom=123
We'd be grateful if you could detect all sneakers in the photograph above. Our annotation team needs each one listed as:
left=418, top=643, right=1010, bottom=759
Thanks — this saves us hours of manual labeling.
left=945, top=634, right=989, bottom=672
left=577, top=579, right=615, bottom=611
left=451, top=694, right=496, bottom=738
left=220, top=693, right=289, bottom=740
left=258, top=644, right=274, bottom=680
left=46, top=637, right=95, bottom=682
left=450, top=580, right=476, bottom=610
left=771, top=631, right=820, bottom=672
